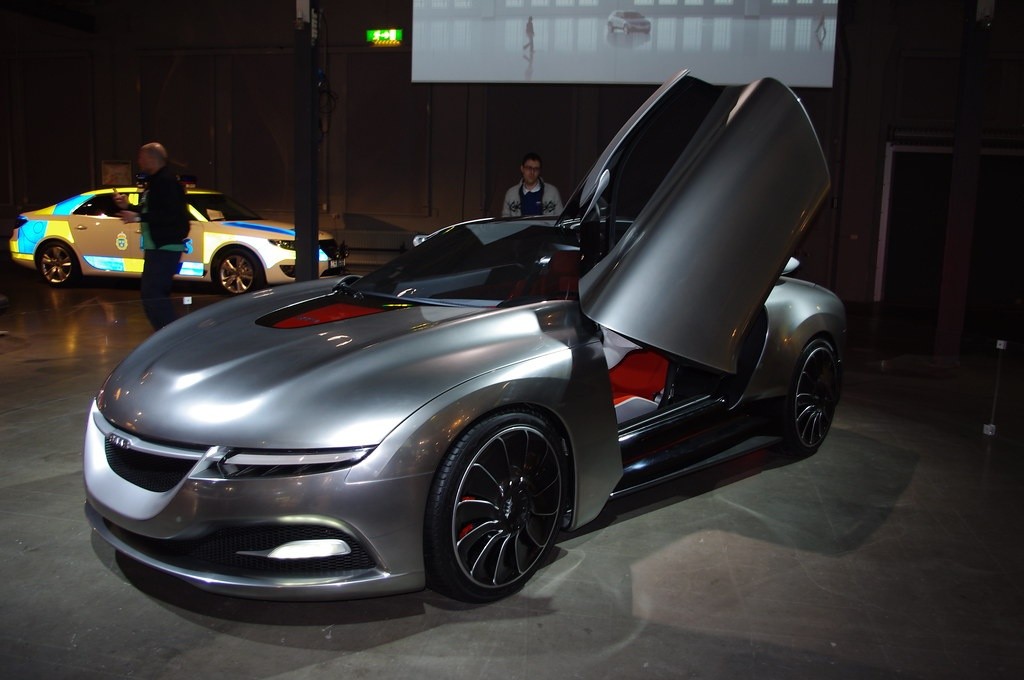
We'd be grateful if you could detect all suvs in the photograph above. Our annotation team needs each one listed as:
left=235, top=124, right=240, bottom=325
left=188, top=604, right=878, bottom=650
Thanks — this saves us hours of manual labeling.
left=608, top=10, right=651, bottom=34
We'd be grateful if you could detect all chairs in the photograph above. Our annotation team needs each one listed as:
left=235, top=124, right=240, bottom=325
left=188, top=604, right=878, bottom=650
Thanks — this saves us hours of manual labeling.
left=607, top=347, right=668, bottom=406
left=509, top=251, right=576, bottom=300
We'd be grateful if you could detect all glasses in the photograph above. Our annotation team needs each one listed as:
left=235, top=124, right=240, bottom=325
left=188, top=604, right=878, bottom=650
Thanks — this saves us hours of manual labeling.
left=522, top=165, right=541, bottom=173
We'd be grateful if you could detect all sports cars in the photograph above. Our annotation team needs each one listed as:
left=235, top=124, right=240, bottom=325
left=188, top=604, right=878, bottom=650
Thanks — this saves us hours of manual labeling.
left=85, top=68, right=848, bottom=603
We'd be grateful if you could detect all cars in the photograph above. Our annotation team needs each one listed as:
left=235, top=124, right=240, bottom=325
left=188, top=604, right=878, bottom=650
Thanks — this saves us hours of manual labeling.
left=10, top=186, right=345, bottom=298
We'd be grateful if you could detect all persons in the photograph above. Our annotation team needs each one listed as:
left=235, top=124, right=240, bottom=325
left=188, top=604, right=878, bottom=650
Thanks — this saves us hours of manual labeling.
left=502, top=154, right=564, bottom=219
left=113, top=141, right=191, bottom=330
